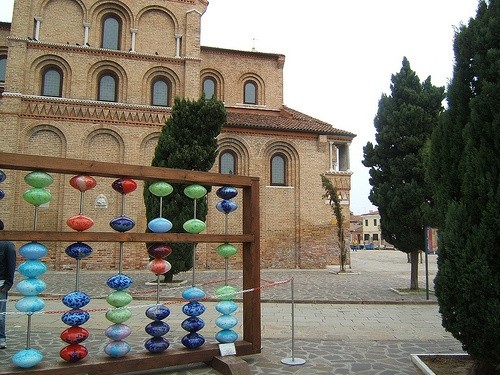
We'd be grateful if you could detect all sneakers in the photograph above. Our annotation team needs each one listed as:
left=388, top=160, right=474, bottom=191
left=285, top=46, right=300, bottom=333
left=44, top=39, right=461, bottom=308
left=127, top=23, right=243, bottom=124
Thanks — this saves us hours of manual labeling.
left=0, top=338, right=7, bottom=348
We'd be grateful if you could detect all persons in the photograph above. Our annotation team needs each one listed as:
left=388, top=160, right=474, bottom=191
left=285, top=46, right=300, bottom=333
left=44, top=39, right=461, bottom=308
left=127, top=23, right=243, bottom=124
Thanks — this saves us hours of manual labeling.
left=0, top=221, right=16, bottom=350
